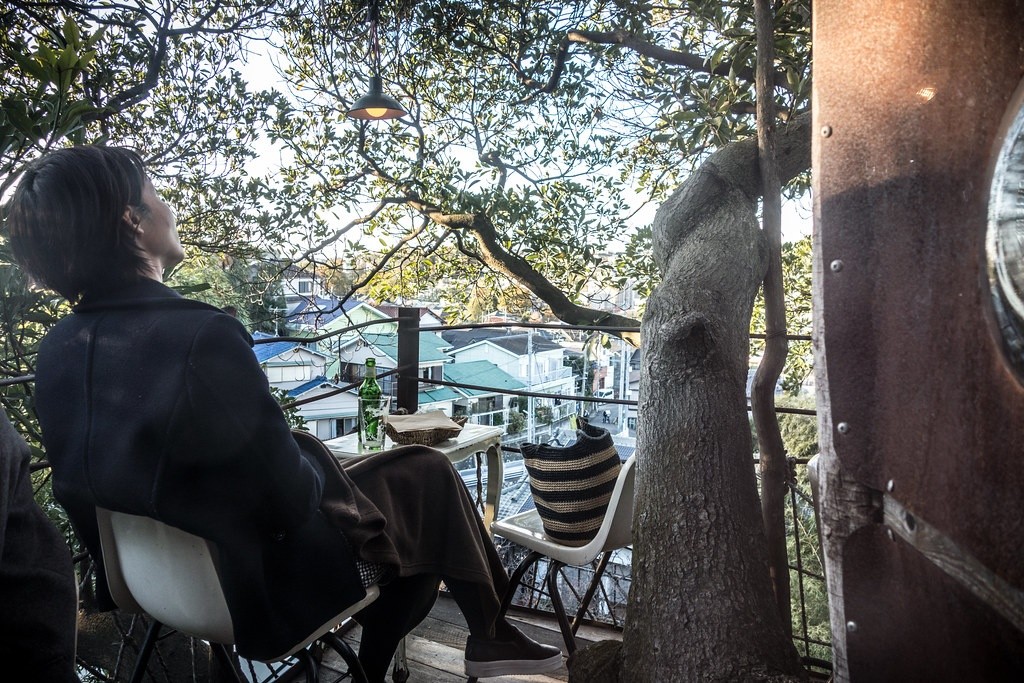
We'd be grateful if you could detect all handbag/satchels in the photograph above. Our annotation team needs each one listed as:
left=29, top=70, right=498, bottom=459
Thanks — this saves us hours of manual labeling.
left=519, top=415, right=622, bottom=549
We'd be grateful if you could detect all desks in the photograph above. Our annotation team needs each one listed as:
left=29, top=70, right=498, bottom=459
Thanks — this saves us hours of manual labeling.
left=319, top=414, right=504, bottom=683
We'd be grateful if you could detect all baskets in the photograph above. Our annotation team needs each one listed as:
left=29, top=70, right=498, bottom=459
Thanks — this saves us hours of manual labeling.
left=384, top=411, right=468, bottom=445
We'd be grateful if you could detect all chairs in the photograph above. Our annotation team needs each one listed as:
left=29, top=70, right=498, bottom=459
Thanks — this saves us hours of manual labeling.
left=94, top=503, right=382, bottom=683
left=490, top=450, right=636, bottom=658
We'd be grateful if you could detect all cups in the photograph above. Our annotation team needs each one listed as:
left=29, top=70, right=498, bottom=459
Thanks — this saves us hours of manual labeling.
left=357, top=396, right=391, bottom=456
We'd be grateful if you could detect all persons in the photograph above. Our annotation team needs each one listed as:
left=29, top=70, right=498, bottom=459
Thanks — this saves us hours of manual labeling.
left=0, top=145, right=566, bottom=683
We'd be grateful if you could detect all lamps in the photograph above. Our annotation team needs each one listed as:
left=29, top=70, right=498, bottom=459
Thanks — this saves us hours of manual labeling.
left=345, top=0, right=408, bottom=120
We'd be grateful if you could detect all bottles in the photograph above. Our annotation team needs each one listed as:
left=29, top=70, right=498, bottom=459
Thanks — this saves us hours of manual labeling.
left=358, top=357, right=381, bottom=450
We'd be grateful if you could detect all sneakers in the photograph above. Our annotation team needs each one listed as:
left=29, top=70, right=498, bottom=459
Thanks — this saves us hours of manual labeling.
left=464, top=623, right=564, bottom=678
